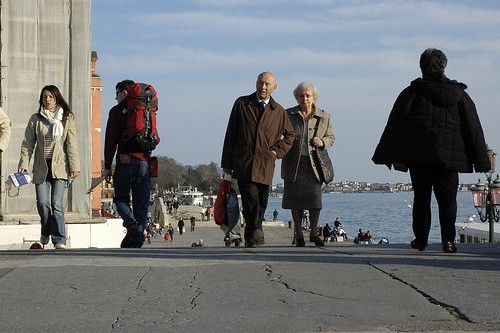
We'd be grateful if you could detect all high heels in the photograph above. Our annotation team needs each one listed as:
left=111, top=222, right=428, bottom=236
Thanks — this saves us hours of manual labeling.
left=295, top=234, right=305, bottom=246
left=309, top=234, right=325, bottom=246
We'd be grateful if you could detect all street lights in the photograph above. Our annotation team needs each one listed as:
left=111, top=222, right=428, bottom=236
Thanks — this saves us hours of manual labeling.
left=466, top=143, right=500, bottom=243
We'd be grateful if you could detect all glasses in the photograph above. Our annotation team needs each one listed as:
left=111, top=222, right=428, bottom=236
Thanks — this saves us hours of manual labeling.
left=116, top=91, right=123, bottom=97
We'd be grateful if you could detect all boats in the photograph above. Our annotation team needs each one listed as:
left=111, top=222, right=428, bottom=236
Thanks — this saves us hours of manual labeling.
left=173, top=182, right=204, bottom=207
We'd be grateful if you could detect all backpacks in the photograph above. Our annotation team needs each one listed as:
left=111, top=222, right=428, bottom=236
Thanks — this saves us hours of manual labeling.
left=116, top=83, right=161, bottom=155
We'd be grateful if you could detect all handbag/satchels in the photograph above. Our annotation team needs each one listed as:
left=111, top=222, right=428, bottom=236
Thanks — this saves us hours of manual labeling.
left=213, top=172, right=241, bottom=240
left=182, top=226, right=186, bottom=232
left=165, top=231, right=170, bottom=240
left=176, top=226, right=180, bottom=232
left=314, top=148, right=334, bottom=185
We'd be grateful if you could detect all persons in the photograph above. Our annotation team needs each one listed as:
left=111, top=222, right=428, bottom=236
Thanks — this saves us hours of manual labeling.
left=303, top=211, right=309, bottom=229
left=191, top=215, right=195, bottom=231
left=18, top=85, right=80, bottom=249
left=324, top=218, right=347, bottom=242
left=169, top=200, right=180, bottom=214
left=178, top=218, right=184, bottom=235
left=200, top=206, right=210, bottom=221
left=192, top=240, right=204, bottom=247
left=356, top=229, right=371, bottom=243
left=147, top=223, right=173, bottom=243
left=371, top=48, right=491, bottom=253
left=273, top=209, right=278, bottom=220
left=281, top=81, right=335, bottom=245
left=104, top=80, right=150, bottom=248
left=0, top=107, right=12, bottom=152
left=221, top=72, right=295, bottom=247
left=30, top=243, right=42, bottom=249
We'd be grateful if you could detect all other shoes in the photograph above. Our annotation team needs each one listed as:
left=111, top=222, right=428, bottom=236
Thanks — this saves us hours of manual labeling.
left=443, top=241, right=458, bottom=253
left=120, top=223, right=146, bottom=248
left=245, top=239, right=257, bottom=248
left=254, top=228, right=264, bottom=244
left=54, top=243, right=66, bottom=249
left=40, top=234, right=50, bottom=244
left=411, top=238, right=425, bottom=251
